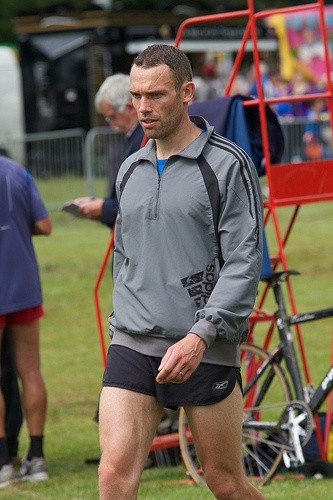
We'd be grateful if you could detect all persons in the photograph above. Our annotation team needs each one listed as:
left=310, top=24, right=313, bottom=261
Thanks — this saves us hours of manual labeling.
left=0, top=147, right=52, bottom=486
left=98, top=45, right=265, bottom=500
left=189, top=20, right=333, bottom=166
left=75, top=74, right=152, bottom=472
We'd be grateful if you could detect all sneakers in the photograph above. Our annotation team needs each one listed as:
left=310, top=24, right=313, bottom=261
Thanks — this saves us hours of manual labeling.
left=19, top=455, right=49, bottom=483
left=0, top=463, right=19, bottom=489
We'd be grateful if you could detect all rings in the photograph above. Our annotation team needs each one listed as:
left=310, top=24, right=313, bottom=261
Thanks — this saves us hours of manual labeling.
left=179, top=372, right=185, bottom=376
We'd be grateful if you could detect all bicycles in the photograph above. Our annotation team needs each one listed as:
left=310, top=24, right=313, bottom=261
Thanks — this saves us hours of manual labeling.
left=179, top=270, right=333, bottom=489
